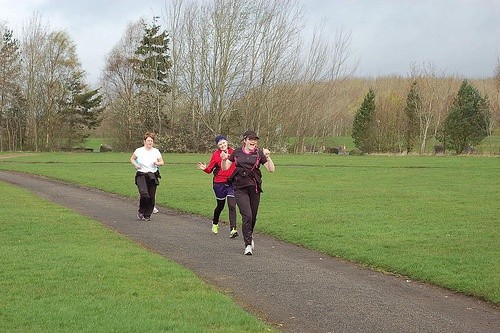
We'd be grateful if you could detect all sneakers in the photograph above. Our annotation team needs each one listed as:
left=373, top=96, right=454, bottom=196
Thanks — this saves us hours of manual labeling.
left=144, top=215, right=151, bottom=221
left=137, top=209, right=144, bottom=220
left=212, top=219, right=218, bottom=233
left=230, top=229, right=239, bottom=238
left=244, top=245, right=253, bottom=255
left=153, top=207, right=158, bottom=214
left=251, top=240, right=254, bottom=250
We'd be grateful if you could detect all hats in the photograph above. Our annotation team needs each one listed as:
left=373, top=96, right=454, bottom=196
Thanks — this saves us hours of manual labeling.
left=216, top=136, right=225, bottom=142
left=243, top=131, right=259, bottom=140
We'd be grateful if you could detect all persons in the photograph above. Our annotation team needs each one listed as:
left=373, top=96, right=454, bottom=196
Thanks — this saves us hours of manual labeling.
left=197, top=136, right=239, bottom=238
left=220, top=131, right=275, bottom=255
left=129, top=132, right=165, bottom=223
